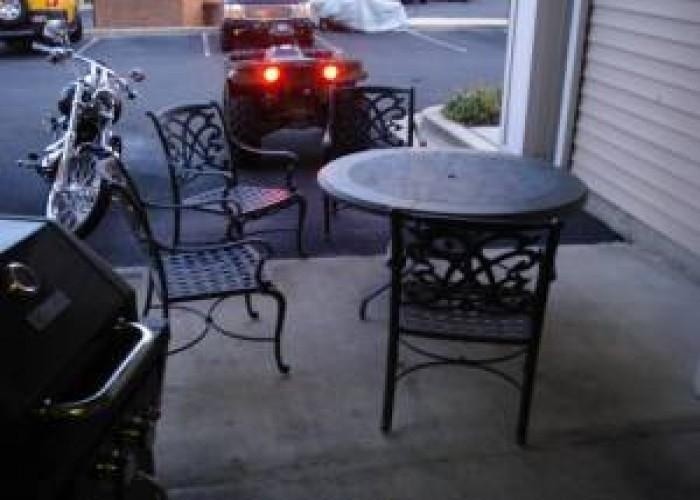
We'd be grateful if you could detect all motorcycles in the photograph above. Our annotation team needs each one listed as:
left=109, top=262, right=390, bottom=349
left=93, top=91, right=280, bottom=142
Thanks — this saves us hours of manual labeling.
left=226, top=47, right=371, bottom=160
left=16, top=17, right=145, bottom=238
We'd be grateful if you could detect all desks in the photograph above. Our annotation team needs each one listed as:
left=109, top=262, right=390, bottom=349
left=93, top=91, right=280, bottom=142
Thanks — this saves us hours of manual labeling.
left=317, top=146, right=589, bottom=322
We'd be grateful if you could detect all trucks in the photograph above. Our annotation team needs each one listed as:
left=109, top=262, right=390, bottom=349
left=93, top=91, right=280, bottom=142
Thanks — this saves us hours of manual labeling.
left=220, top=0, right=315, bottom=50
left=1, top=0, right=82, bottom=56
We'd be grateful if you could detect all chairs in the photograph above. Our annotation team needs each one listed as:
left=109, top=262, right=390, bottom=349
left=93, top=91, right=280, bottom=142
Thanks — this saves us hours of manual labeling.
left=144, top=99, right=311, bottom=261
left=96, top=151, right=293, bottom=375
left=321, top=84, right=415, bottom=237
left=378, top=207, right=560, bottom=446
